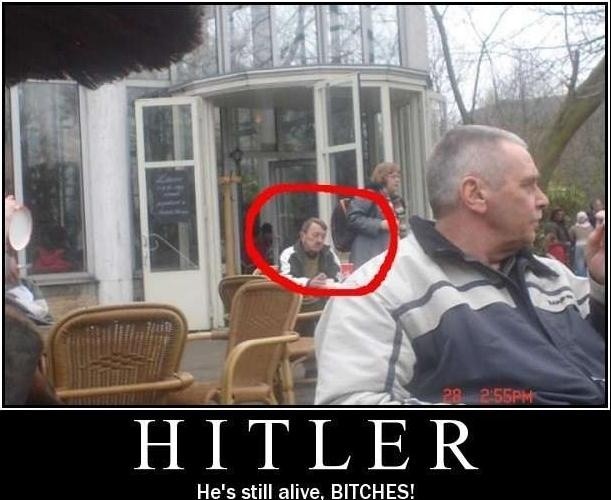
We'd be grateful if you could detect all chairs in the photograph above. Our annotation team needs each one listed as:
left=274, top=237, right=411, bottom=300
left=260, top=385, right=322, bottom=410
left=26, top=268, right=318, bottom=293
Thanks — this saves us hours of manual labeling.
left=5, top=275, right=325, bottom=404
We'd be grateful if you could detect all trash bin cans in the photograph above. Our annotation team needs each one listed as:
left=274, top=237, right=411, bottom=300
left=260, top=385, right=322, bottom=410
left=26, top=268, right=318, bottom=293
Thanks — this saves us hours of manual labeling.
left=548, top=244, right=568, bottom=266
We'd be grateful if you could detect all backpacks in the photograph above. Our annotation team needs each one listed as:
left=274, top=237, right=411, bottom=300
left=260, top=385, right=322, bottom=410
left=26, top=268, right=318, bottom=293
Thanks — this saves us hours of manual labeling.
left=331, top=198, right=357, bottom=252
left=551, top=244, right=568, bottom=261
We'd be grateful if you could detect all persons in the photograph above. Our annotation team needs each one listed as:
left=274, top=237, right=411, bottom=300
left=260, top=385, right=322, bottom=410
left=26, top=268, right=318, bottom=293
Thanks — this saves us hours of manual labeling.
left=5, top=246, right=52, bottom=405
left=240, top=217, right=344, bottom=289
left=313, top=123, right=605, bottom=407
left=35, top=222, right=80, bottom=272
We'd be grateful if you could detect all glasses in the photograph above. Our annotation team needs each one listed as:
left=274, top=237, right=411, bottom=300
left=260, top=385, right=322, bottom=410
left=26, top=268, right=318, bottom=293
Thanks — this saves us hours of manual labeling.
left=387, top=175, right=402, bottom=180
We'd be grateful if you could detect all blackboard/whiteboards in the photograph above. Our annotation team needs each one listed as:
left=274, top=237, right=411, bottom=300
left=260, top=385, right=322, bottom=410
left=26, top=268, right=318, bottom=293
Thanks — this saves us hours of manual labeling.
left=149, top=170, right=189, bottom=219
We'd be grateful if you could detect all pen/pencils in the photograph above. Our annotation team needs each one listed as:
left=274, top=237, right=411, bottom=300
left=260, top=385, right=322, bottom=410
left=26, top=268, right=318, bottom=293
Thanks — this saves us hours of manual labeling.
left=16, top=265, right=33, bottom=268
